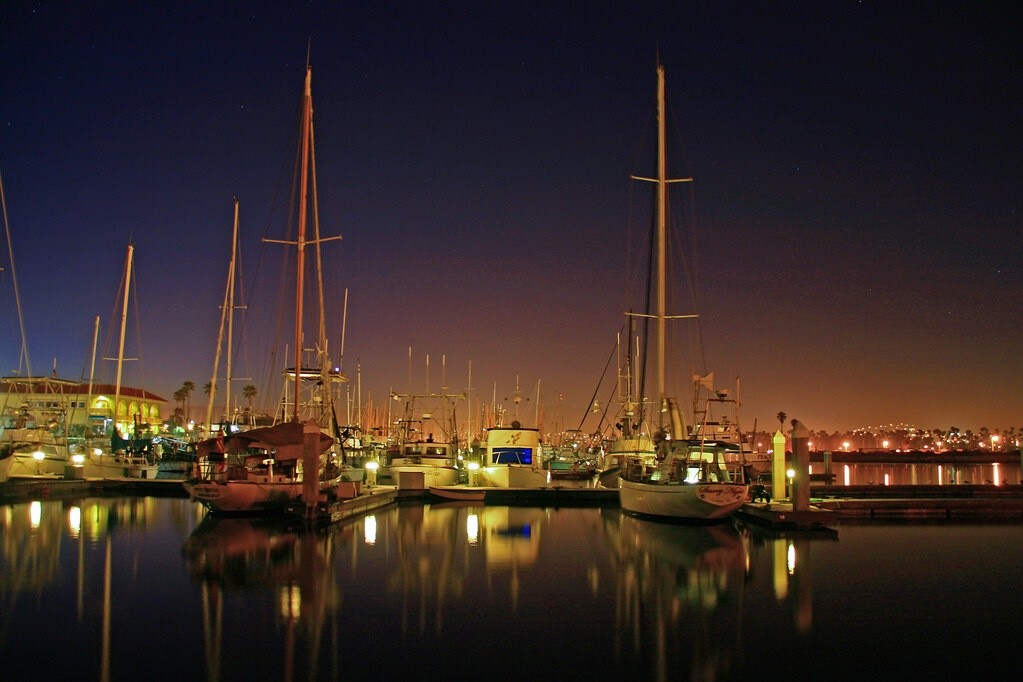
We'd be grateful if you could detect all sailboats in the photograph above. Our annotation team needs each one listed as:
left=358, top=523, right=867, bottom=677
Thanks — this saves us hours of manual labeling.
left=600, top=505, right=752, bottom=682
left=179, top=508, right=361, bottom=682
left=2, top=59, right=753, bottom=528
